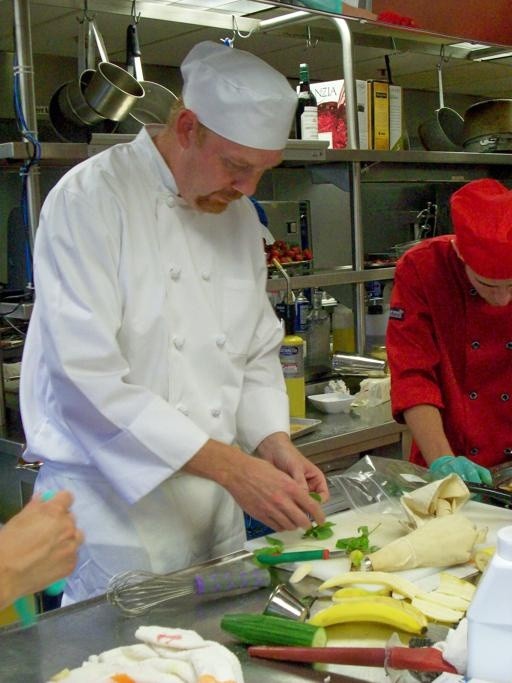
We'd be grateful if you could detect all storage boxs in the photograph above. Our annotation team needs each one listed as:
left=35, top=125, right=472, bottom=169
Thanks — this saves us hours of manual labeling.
left=296, top=79, right=404, bottom=151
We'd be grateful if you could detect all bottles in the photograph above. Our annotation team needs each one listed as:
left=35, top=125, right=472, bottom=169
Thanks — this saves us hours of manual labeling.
left=293, top=61, right=321, bottom=143
left=306, top=291, right=331, bottom=370
left=279, top=334, right=306, bottom=420
left=463, top=528, right=512, bottom=683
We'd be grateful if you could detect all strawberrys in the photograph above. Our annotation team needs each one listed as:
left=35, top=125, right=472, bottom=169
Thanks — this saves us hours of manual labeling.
left=262, top=239, right=316, bottom=266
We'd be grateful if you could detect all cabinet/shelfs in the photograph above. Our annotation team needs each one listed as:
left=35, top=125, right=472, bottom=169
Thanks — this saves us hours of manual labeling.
left=0, top=0, right=512, bottom=437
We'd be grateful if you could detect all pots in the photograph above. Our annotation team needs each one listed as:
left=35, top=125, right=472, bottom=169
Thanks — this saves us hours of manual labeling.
left=47, top=16, right=182, bottom=143
left=418, top=62, right=512, bottom=154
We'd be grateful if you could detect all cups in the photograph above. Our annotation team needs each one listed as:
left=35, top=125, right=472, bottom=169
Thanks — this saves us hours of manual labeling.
left=260, top=582, right=308, bottom=624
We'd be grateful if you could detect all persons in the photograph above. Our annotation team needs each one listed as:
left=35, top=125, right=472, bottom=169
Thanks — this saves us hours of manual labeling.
left=1, top=487, right=84, bottom=608
left=21, top=41, right=331, bottom=609
left=385, top=178, right=512, bottom=484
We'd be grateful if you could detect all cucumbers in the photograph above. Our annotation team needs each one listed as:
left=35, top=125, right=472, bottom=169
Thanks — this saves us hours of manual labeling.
left=216, top=611, right=328, bottom=649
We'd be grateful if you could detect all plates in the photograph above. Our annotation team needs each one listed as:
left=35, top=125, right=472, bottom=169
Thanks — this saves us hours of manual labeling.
left=306, top=392, right=357, bottom=416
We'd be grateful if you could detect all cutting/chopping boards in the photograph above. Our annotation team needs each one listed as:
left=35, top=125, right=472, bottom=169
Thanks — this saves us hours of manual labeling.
left=244, top=492, right=512, bottom=593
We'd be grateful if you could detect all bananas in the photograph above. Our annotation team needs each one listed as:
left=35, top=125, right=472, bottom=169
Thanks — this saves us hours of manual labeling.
left=311, top=600, right=427, bottom=634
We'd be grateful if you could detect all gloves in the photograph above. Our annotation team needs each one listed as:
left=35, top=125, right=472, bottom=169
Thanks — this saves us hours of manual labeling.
left=427, top=455, right=493, bottom=486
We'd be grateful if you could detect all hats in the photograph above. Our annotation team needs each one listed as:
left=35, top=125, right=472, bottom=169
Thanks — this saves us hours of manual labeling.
left=180, top=40, right=299, bottom=150
left=448, top=178, right=512, bottom=280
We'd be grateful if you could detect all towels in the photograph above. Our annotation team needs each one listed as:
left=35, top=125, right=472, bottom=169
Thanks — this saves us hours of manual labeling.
left=365, top=472, right=489, bottom=576
left=45, top=625, right=244, bottom=683
